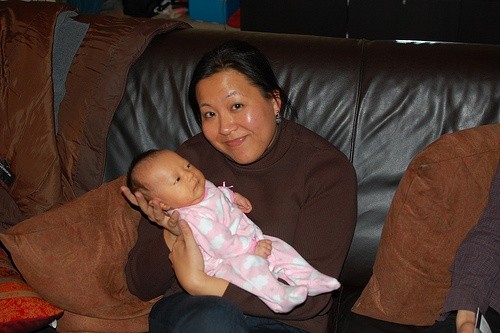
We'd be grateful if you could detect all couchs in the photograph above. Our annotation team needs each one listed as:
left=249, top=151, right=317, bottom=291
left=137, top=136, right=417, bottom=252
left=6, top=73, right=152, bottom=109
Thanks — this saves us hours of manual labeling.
left=0, top=0, right=500, bottom=333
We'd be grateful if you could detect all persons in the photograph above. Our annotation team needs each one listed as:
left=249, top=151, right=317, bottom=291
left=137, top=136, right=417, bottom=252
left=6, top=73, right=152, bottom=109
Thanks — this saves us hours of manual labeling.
left=440, top=162, right=500, bottom=333
left=120, top=40, right=357, bottom=333
left=125, top=149, right=341, bottom=313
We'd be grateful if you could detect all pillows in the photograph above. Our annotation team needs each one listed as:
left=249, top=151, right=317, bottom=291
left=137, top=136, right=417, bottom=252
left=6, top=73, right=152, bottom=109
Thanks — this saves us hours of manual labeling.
left=0, top=176, right=164, bottom=333
left=0, top=247, right=63, bottom=333
left=350, top=124, right=500, bottom=326
